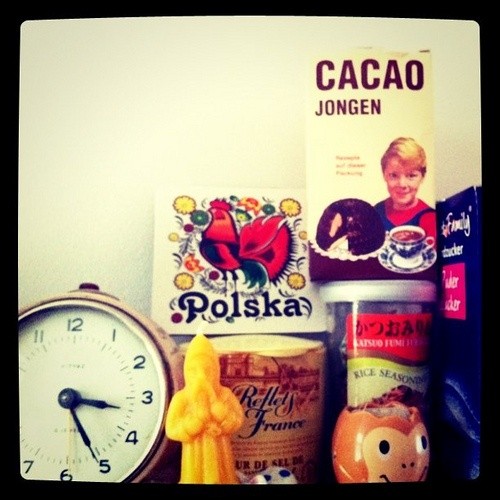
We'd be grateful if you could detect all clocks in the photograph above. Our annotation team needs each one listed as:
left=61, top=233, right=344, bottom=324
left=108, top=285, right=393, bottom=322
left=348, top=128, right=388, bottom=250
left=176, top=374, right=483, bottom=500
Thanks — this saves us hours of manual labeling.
left=19, top=281, right=186, bottom=485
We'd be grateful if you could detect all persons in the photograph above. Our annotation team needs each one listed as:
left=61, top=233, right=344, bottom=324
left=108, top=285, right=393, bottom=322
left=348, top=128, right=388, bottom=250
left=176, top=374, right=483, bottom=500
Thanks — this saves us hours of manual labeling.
left=165, top=333, right=243, bottom=484
left=375, top=135, right=436, bottom=250
